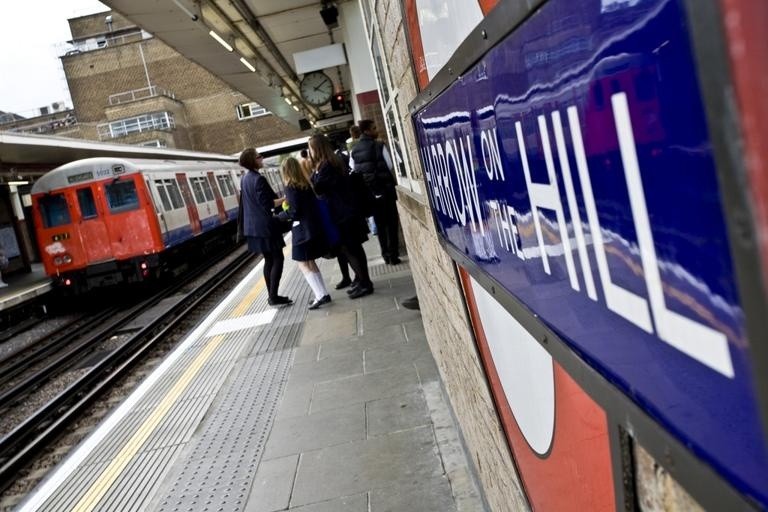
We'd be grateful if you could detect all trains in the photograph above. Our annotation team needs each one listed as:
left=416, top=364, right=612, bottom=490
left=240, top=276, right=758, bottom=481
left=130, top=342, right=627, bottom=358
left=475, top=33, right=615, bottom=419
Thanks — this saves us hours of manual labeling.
left=30, top=151, right=284, bottom=303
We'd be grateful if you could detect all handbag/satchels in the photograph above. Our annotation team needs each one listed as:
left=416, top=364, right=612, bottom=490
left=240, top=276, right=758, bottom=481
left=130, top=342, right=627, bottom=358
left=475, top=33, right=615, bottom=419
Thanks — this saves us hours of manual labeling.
left=348, top=166, right=377, bottom=217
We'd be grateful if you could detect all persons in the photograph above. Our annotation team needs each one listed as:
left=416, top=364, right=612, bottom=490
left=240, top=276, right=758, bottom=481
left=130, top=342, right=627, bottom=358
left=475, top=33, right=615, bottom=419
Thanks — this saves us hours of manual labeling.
left=239, top=120, right=420, bottom=309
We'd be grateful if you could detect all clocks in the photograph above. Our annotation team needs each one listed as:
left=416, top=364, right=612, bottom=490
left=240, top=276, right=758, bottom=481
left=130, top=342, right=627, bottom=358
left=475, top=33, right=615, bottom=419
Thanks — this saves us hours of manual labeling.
left=299, top=71, right=334, bottom=106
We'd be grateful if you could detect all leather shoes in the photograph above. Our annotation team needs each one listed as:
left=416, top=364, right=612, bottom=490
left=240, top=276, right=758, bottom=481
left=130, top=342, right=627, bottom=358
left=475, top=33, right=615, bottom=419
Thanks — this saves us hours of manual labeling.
left=335, top=278, right=373, bottom=299
left=268, top=297, right=293, bottom=305
left=309, top=295, right=331, bottom=309
